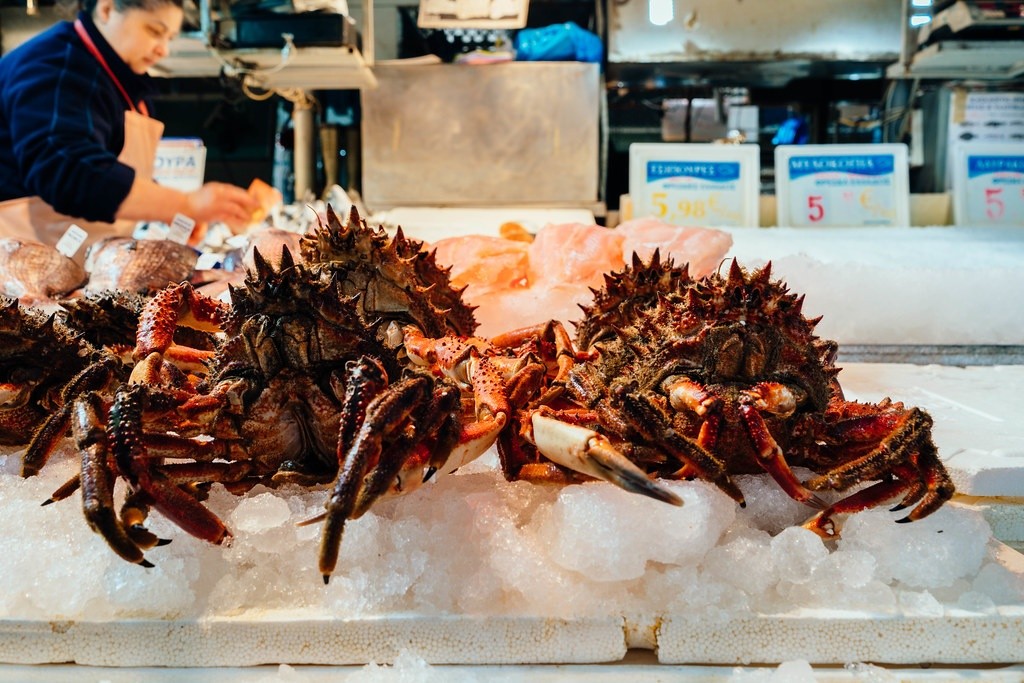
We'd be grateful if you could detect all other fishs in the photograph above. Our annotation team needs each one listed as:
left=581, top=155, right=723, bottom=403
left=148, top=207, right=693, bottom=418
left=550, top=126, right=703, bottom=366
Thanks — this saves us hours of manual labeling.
left=0, top=238, right=88, bottom=316
left=82, top=235, right=200, bottom=298
left=413, top=214, right=735, bottom=295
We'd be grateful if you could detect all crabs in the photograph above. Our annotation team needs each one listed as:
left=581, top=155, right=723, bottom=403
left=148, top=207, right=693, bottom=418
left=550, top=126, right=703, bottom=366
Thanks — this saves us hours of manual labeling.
left=194, top=203, right=684, bottom=585
left=0, top=287, right=228, bottom=568
left=496, top=248, right=957, bottom=542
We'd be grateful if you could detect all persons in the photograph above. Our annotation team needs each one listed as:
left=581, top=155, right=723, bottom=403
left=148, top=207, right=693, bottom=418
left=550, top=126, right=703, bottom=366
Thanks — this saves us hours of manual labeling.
left=0, top=0, right=254, bottom=226
